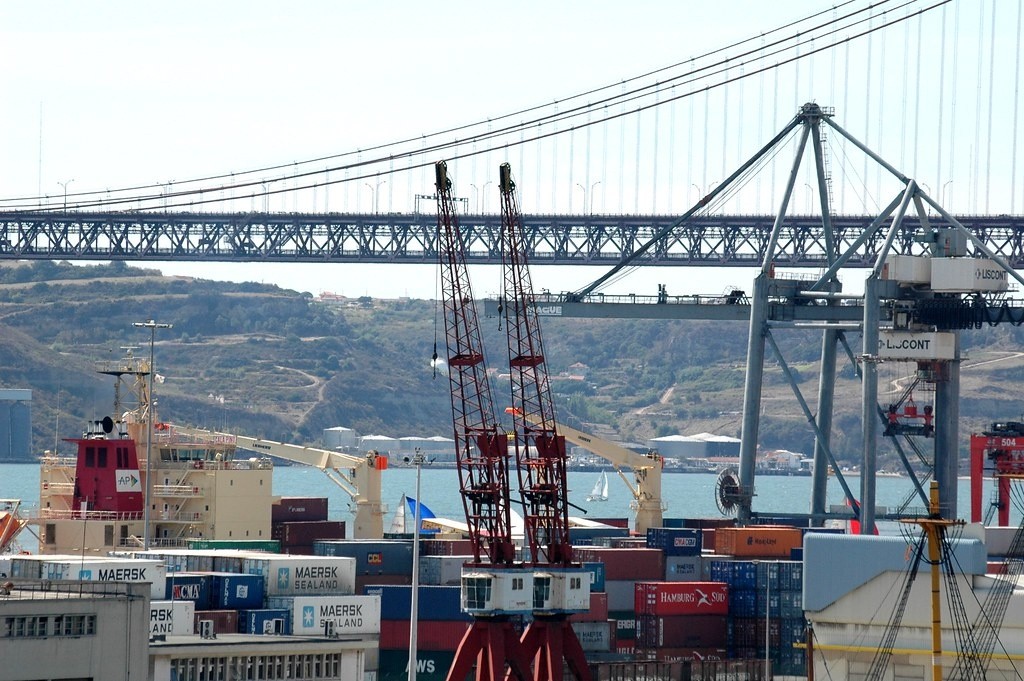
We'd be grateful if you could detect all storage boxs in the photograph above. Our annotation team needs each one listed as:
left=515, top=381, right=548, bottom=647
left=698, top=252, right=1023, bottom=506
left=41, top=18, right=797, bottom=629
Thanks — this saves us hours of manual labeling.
left=874, top=228, right=1009, bottom=359
left=0, top=496, right=808, bottom=681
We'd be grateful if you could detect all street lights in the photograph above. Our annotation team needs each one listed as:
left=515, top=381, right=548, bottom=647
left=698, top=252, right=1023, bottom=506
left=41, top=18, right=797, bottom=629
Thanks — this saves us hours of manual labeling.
left=132, top=318, right=171, bottom=554
left=403, top=446, right=435, bottom=681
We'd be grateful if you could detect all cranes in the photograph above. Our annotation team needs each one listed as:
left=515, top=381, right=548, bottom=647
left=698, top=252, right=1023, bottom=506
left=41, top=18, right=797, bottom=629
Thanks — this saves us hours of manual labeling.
left=497, top=161, right=590, bottom=619
left=428, top=158, right=533, bottom=620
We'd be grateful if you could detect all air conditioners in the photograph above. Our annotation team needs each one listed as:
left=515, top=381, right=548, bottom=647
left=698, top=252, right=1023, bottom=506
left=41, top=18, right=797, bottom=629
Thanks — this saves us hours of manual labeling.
left=325, top=620, right=335, bottom=635
left=199, top=620, right=213, bottom=638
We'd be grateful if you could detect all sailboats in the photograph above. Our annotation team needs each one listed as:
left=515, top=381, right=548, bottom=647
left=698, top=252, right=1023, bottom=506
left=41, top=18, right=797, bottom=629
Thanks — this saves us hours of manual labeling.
left=586, top=467, right=608, bottom=502
